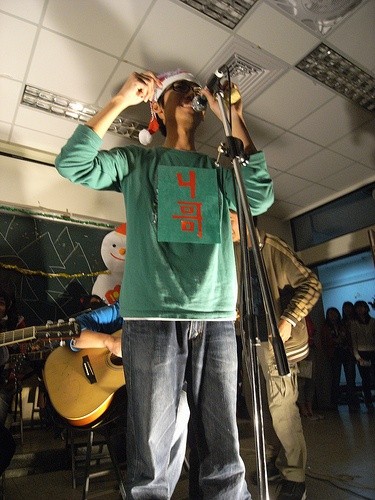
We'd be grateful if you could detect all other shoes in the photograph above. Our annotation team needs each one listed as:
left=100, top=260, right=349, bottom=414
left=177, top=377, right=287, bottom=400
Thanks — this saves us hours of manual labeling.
left=308, top=410, right=326, bottom=420
left=254, top=461, right=282, bottom=481
left=349, top=403, right=359, bottom=410
left=273, top=479, right=306, bottom=500
left=365, top=399, right=374, bottom=410
left=302, top=413, right=319, bottom=421
left=328, top=401, right=337, bottom=409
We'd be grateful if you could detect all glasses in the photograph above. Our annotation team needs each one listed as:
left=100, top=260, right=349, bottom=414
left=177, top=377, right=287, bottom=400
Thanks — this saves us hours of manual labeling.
left=161, top=79, right=200, bottom=96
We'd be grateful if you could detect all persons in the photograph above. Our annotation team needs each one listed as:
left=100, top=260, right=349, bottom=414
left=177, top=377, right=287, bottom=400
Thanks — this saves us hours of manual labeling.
left=54, top=71, right=274, bottom=500
left=298, top=300, right=375, bottom=422
left=0, top=295, right=128, bottom=479
left=229, top=208, right=322, bottom=500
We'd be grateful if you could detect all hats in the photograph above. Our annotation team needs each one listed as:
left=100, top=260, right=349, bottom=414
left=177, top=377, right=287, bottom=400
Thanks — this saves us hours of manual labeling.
left=139, top=70, right=203, bottom=147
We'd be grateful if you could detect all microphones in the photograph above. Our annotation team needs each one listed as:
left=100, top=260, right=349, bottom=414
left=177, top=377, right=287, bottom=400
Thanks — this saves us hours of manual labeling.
left=191, top=67, right=223, bottom=113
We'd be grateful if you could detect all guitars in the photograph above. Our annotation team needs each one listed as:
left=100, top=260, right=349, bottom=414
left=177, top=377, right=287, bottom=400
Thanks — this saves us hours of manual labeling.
left=0, top=317, right=81, bottom=347
left=43, top=328, right=126, bottom=426
